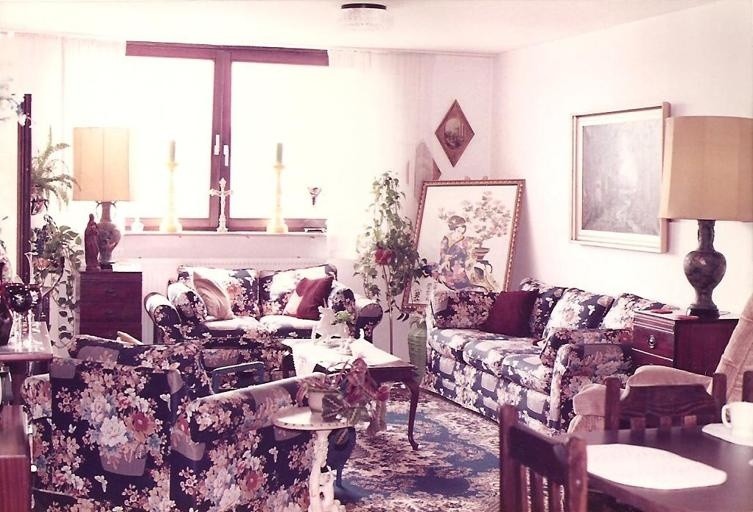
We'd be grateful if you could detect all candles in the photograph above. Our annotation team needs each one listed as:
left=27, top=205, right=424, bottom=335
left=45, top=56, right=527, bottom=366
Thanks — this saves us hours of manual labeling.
left=276, top=141, right=282, bottom=164
left=169, top=138, right=176, bottom=164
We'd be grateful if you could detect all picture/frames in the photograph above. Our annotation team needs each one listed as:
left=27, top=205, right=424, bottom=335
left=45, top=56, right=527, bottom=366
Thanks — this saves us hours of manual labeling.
left=570, top=103, right=671, bottom=254
left=400, top=179, right=525, bottom=312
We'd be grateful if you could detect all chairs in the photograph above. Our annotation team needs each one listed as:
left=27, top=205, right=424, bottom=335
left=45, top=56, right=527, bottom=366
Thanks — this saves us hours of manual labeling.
left=742, top=371, right=753, bottom=404
left=603, top=373, right=727, bottom=428
left=500, top=405, right=587, bottom=512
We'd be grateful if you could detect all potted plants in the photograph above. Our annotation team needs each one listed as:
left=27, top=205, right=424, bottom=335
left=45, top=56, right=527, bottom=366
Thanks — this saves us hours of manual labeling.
left=19, top=215, right=83, bottom=354
left=298, top=359, right=389, bottom=437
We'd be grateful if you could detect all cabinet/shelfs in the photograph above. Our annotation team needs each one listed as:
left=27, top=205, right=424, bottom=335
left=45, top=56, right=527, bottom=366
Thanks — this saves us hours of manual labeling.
left=0, top=313, right=53, bottom=510
left=79, top=264, right=143, bottom=344
left=633, top=309, right=738, bottom=375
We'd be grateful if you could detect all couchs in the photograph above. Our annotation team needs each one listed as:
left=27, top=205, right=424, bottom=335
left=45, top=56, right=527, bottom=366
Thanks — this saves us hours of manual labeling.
left=144, top=265, right=382, bottom=376
left=20, top=357, right=331, bottom=512
left=419, top=278, right=686, bottom=441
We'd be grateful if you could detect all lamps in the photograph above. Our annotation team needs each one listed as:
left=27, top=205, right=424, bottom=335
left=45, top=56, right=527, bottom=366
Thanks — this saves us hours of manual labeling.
left=659, top=114, right=753, bottom=318
left=73, top=126, right=132, bottom=266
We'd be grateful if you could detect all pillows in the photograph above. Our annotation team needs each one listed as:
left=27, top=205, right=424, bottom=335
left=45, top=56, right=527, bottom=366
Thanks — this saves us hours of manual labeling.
left=540, top=328, right=632, bottom=369
left=283, top=278, right=332, bottom=320
left=478, top=287, right=541, bottom=338
left=192, top=271, right=234, bottom=320
left=67, top=334, right=213, bottom=397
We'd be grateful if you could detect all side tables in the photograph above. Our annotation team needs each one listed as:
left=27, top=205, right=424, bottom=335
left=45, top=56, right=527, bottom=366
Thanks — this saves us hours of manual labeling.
left=273, top=407, right=354, bottom=512
